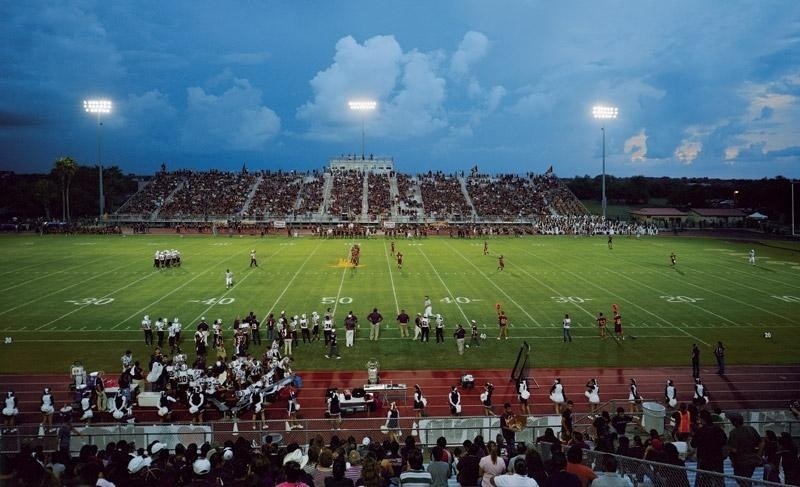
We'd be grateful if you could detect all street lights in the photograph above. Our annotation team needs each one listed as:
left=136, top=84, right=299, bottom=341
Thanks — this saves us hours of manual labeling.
left=347, top=99, right=379, bottom=158
left=590, top=104, right=620, bottom=219
left=82, top=98, right=113, bottom=220
left=734, top=189, right=739, bottom=203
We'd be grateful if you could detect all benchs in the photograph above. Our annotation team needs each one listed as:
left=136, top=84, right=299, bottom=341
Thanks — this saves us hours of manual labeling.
left=136, top=391, right=161, bottom=407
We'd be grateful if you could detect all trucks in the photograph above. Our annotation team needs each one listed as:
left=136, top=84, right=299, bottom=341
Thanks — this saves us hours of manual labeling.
left=36, top=220, right=67, bottom=234
left=0, top=219, right=22, bottom=232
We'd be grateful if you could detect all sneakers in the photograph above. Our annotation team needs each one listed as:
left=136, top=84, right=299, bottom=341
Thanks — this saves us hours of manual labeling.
left=290, top=424, right=303, bottom=429
left=262, top=425, right=269, bottom=429
left=588, top=414, right=601, bottom=420
left=633, top=416, right=640, bottom=420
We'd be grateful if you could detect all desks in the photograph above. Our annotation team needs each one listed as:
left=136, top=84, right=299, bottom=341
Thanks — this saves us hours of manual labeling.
left=105, top=384, right=139, bottom=403
left=364, top=383, right=407, bottom=405
left=337, top=393, right=373, bottom=417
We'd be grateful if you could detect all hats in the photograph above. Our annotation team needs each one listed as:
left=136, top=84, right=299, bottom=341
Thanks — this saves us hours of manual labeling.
left=223, top=448, right=233, bottom=460
left=283, top=449, right=309, bottom=470
left=127, top=456, right=152, bottom=474
left=650, top=437, right=664, bottom=449
left=349, top=449, right=361, bottom=464
left=362, top=437, right=370, bottom=445
left=79, top=444, right=98, bottom=460
left=151, top=442, right=167, bottom=454
left=193, top=457, right=211, bottom=475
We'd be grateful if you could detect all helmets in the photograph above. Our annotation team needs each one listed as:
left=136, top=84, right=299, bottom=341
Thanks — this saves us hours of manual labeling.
left=201, top=317, right=205, bottom=321
left=217, top=319, right=223, bottom=323
left=312, top=311, right=318, bottom=317
left=294, top=315, right=298, bottom=320
left=301, top=314, right=307, bottom=319
left=144, top=315, right=149, bottom=320
left=164, top=318, right=168, bottom=323
left=174, top=318, right=179, bottom=322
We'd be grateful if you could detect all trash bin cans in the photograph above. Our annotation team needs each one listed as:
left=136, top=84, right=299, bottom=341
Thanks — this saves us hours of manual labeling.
left=643, top=402, right=666, bottom=436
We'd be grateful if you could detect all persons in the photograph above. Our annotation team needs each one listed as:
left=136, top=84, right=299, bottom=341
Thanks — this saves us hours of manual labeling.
left=596, top=312, right=607, bottom=340
left=713, top=341, right=725, bottom=374
left=396, top=252, right=403, bottom=270
left=607, top=235, right=612, bottom=248
left=496, top=255, right=504, bottom=271
left=562, top=313, right=572, bottom=343
left=153, top=248, right=181, bottom=268
left=483, top=241, right=489, bottom=255
left=496, top=304, right=509, bottom=340
left=351, top=244, right=360, bottom=266
left=390, top=241, right=395, bottom=257
left=613, top=304, right=625, bottom=340
left=690, top=343, right=701, bottom=378
left=1, top=166, right=800, bottom=240
left=249, top=249, right=258, bottom=268
left=226, top=268, right=233, bottom=290
left=670, top=252, right=676, bottom=265
left=140, top=295, right=482, bottom=362
left=749, top=249, right=755, bottom=266
left=1, top=350, right=800, bottom=487
